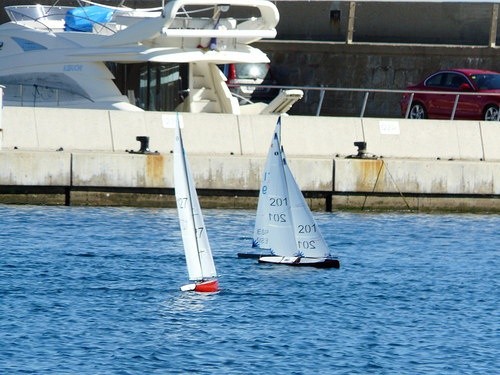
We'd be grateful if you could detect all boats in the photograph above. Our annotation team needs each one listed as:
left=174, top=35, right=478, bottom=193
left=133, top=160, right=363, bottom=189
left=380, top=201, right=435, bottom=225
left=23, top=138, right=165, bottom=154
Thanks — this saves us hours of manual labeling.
left=0, top=0, right=303, bottom=117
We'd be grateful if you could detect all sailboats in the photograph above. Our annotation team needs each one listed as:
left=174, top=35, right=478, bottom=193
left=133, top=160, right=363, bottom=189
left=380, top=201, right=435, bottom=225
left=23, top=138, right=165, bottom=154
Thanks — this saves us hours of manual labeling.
left=237, top=115, right=340, bottom=267
left=172, top=113, right=221, bottom=292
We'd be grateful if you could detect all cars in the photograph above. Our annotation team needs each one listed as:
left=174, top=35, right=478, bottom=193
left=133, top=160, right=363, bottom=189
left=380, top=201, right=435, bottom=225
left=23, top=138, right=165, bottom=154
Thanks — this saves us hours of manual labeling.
left=399, top=68, right=500, bottom=121
